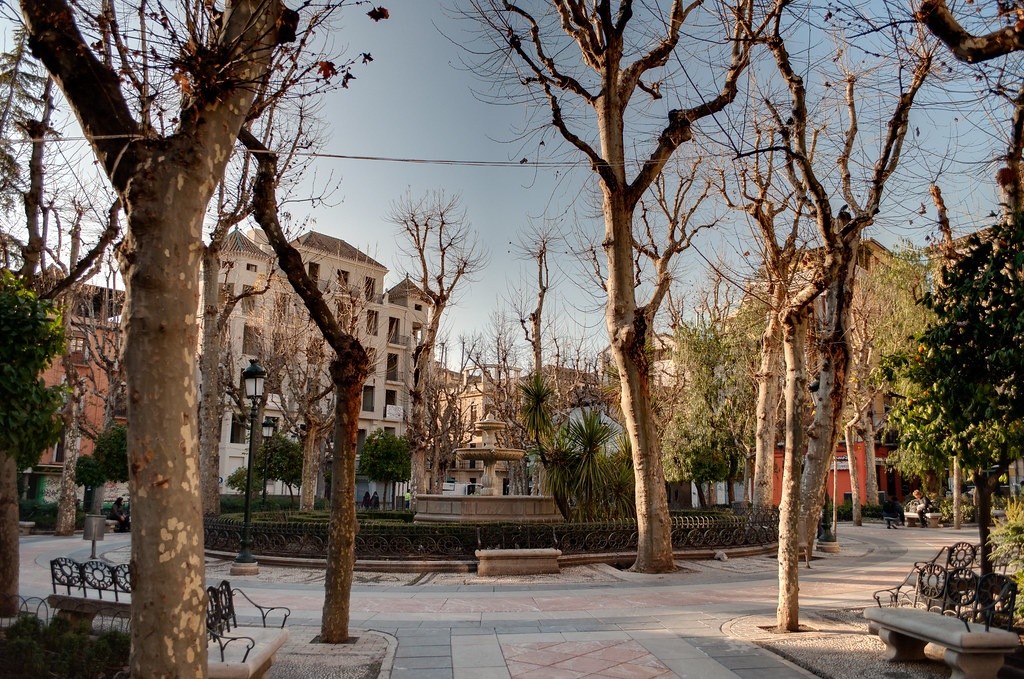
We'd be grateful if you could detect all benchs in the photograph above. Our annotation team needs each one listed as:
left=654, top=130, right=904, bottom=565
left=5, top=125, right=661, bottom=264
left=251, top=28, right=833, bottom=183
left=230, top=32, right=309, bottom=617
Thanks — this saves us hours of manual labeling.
left=904, top=499, right=943, bottom=528
left=474, top=524, right=561, bottom=576
left=19, top=504, right=35, bottom=536
left=863, top=542, right=1019, bottom=679
left=48, top=557, right=130, bottom=630
left=101, top=508, right=119, bottom=533
left=207, top=580, right=290, bottom=679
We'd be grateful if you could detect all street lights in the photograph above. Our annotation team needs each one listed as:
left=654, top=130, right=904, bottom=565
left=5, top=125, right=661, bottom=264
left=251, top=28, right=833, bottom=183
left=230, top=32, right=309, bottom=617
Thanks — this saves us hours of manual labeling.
left=228, top=358, right=270, bottom=563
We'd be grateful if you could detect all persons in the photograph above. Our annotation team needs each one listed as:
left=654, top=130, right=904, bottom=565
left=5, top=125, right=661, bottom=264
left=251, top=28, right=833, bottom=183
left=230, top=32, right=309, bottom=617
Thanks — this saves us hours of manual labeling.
left=913, top=490, right=934, bottom=528
left=361, top=491, right=379, bottom=509
left=110, top=497, right=129, bottom=532
left=882, top=496, right=905, bottom=529
left=405, top=489, right=410, bottom=508
